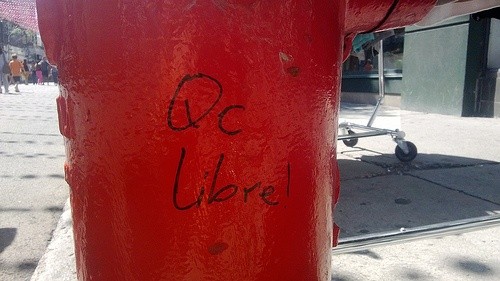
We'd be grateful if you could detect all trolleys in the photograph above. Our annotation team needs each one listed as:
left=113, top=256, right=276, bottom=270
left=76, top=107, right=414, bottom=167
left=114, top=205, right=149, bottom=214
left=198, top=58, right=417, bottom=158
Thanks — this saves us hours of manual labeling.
left=337, top=27, right=418, bottom=161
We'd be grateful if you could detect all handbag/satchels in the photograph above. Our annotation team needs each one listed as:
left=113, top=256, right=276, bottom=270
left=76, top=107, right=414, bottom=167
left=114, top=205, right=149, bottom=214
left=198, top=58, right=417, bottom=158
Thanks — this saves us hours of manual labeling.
left=2, top=64, right=11, bottom=74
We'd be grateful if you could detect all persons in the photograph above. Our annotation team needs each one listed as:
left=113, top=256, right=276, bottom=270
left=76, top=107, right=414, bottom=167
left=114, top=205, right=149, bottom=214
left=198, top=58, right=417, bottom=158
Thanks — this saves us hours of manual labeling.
left=8, top=53, right=25, bottom=92
left=0, top=47, right=11, bottom=93
left=1, top=57, right=60, bottom=86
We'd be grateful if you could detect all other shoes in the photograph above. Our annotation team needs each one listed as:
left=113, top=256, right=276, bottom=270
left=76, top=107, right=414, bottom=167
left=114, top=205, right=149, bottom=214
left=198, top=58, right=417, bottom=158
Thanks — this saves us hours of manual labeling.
left=15, top=90, right=20, bottom=92
